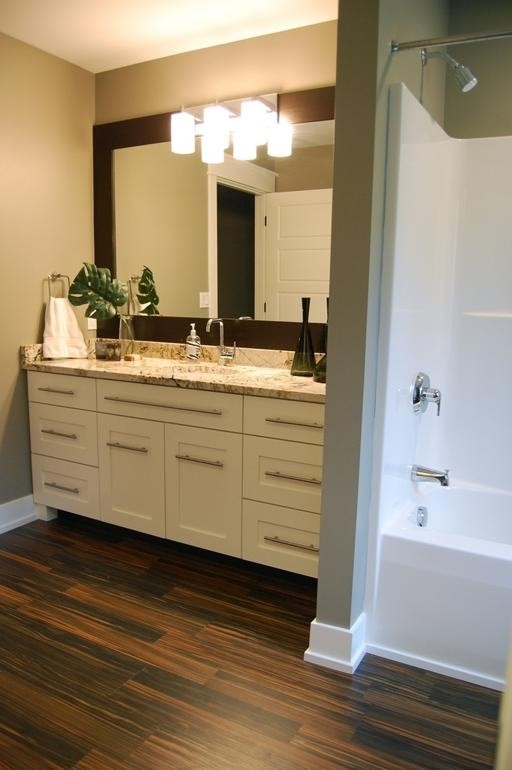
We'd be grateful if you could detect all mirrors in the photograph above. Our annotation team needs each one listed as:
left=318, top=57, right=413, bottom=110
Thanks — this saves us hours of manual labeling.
left=92, top=83, right=335, bottom=354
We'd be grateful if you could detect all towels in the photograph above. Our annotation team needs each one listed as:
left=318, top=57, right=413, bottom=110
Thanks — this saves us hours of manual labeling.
left=42, top=296, right=88, bottom=358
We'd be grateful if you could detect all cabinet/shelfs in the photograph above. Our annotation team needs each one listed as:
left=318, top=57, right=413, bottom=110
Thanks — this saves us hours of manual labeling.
left=95, top=371, right=243, bottom=559
left=28, top=369, right=100, bottom=524
left=243, top=393, right=325, bottom=580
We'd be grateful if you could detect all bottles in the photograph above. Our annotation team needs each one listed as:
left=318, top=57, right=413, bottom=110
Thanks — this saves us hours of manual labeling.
left=290, top=297, right=329, bottom=383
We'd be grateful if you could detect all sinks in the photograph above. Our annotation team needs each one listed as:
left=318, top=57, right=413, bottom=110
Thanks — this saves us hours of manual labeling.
left=154, top=365, right=238, bottom=376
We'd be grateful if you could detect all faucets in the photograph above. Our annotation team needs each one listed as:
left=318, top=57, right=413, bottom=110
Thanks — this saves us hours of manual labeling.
left=410, top=465, right=451, bottom=488
left=239, top=316, right=253, bottom=320
left=206, top=318, right=237, bottom=366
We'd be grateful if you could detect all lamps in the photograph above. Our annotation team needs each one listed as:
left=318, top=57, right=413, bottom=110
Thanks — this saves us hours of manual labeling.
left=170, top=100, right=267, bottom=155
left=201, top=112, right=293, bottom=164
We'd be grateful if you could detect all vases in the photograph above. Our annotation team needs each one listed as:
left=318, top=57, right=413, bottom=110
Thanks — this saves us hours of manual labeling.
left=118, top=316, right=135, bottom=361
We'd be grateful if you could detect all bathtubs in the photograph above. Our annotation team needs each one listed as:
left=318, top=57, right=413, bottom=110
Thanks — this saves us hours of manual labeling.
left=367, top=480, right=511, bottom=691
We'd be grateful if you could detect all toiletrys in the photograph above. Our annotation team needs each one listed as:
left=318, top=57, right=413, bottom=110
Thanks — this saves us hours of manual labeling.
left=185, top=323, right=203, bottom=362
